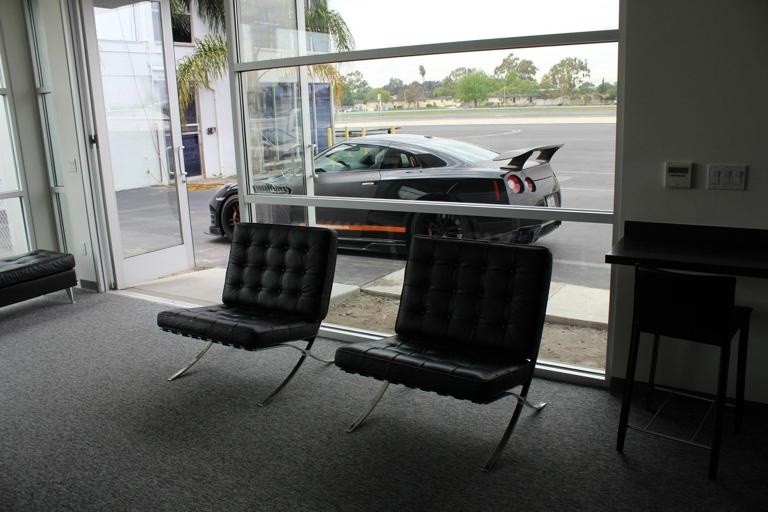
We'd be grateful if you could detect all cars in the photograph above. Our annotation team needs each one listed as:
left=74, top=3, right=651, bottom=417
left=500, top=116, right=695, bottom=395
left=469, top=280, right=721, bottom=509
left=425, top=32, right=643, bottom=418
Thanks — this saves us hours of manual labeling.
left=261, top=127, right=303, bottom=162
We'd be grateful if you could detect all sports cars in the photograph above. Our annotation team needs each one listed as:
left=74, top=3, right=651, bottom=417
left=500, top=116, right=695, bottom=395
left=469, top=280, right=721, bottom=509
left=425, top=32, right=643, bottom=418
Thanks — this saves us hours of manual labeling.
left=209, top=133, right=564, bottom=260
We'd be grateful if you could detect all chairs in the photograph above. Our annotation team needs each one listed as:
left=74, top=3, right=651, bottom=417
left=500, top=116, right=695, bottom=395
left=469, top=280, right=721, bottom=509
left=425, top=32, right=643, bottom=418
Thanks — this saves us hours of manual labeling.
left=334, top=235, right=552, bottom=472
left=616, top=262, right=754, bottom=482
left=157, top=223, right=337, bottom=407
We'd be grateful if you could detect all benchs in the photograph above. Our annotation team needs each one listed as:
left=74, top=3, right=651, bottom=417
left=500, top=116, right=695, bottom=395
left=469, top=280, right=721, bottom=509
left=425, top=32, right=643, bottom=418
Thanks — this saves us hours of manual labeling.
left=0, top=249, right=77, bottom=308
